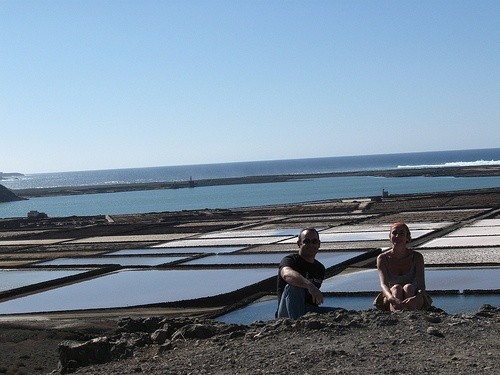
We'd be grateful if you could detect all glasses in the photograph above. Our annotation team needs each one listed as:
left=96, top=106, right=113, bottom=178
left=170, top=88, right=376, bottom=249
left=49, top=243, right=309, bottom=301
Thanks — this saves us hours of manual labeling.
left=299, top=239, right=320, bottom=245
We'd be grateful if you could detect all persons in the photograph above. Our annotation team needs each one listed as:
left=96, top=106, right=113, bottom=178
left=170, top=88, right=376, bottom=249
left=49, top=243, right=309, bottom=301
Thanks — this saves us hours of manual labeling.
left=374, top=222, right=432, bottom=312
left=275, top=227, right=344, bottom=318
